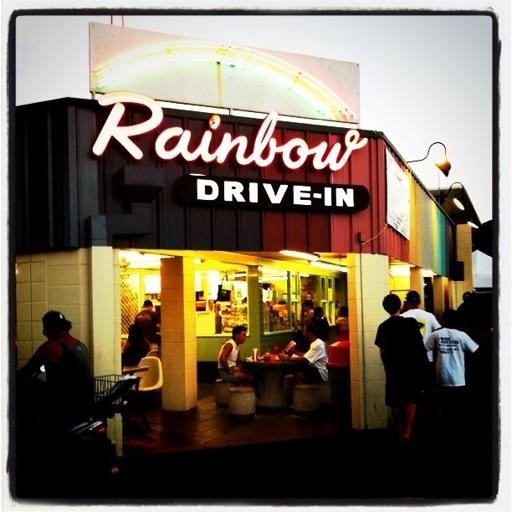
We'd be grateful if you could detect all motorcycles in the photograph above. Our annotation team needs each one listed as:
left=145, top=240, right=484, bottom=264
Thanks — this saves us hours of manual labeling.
left=16, top=369, right=130, bottom=497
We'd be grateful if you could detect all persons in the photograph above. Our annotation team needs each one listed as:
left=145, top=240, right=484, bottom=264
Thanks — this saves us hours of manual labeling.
left=217, top=325, right=255, bottom=387
left=15, top=309, right=93, bottom=444
left=422, top=307, right=482, bottom=402
left=374, top=294, right=428, bottom=445
left=295, top=299, right=314, bottom=331
left=399, top=289, right=444, bottom=364
left=134, top=298, right=161, bottom=343
left=120, top=322, right=150, bottom=368
left=276, top=321, right=329, bottom=405
left=334, top=304, right=348, bottom=338
left=309, top=306, right=328, bottom=340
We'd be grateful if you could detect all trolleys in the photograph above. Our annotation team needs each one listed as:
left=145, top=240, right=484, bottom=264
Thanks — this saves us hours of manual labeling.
left=93, top=366, right=150, bottom=430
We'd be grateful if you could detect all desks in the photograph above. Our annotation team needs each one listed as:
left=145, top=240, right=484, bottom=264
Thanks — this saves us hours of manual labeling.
left=245, top=352, right=303, bottom=412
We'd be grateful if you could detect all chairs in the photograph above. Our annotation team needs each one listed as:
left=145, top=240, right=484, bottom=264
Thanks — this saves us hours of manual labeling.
left=129, top=357, right=163, bottom=393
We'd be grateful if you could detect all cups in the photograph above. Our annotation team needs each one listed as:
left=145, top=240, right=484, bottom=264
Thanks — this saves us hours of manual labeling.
left=253, top=348, right=260, bottom=362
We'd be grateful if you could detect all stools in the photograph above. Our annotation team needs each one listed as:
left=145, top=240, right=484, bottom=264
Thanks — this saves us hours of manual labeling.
left=214, top=377, right=330, bottom=422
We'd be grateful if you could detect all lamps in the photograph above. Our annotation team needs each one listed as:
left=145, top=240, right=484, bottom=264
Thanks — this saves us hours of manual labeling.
left=404, top=140, right=449, bottom=178
